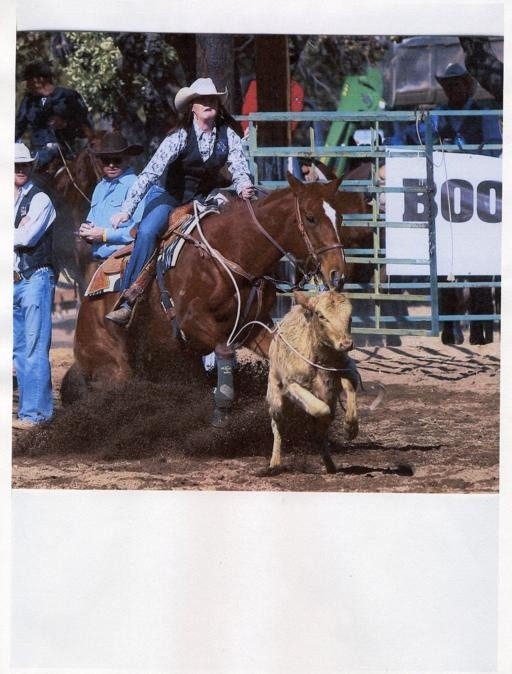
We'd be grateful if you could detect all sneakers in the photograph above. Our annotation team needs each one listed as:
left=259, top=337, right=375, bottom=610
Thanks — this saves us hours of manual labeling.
left=12, top=419, right=45, bottom=430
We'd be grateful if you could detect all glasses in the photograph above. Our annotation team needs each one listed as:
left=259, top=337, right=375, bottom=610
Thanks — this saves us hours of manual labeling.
left=102, top=156, right=123, bottom=165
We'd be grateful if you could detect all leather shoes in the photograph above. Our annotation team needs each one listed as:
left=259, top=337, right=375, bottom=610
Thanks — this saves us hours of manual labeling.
left=104, top=308, right=131, bottom=326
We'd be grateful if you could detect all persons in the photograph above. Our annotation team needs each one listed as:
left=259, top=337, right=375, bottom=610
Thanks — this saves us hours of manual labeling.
left=407, top=63, right=483, bottom=146
left=105, top=77, right=255, bottom=323
left=76, top=134, right=144, bottom=264
left=242, top=48, right=303, bottom=144
left=13, top=141, right=59, bottom=431
left=15, top=63, right=92, bottom=173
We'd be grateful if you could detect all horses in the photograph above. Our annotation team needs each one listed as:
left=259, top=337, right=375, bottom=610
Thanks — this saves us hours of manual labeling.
left=311, top=157, right=493, bottom=346
left=59, top=169, right=349, bottom=429
left=39, top=123, right=110, bottom=299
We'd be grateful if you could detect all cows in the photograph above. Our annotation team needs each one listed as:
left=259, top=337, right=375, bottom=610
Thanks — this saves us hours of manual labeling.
left=265, top=278, right=359, bottom=477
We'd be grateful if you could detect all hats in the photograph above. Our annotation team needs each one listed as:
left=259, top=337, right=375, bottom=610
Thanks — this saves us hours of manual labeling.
left=14, top=143, right=40, bottom=166
left=174, top=78, right=229, bottom=113
left=87, top=132, right=144, bottom=159
left=435, top=62, right=478, bottom=98
left=16, top=62, right=61, bottom=81
